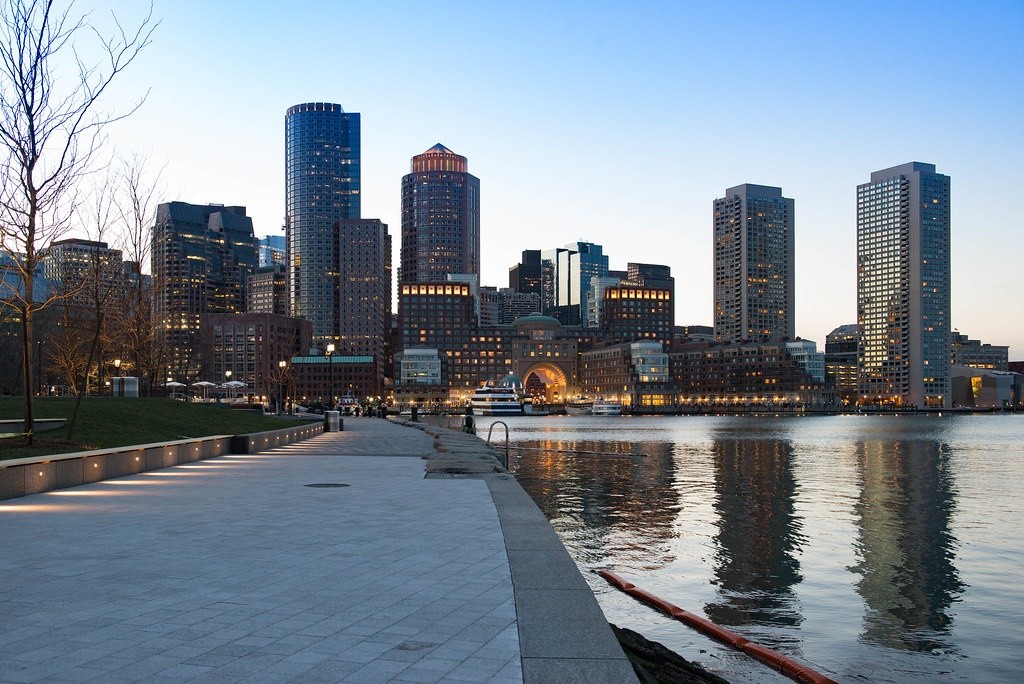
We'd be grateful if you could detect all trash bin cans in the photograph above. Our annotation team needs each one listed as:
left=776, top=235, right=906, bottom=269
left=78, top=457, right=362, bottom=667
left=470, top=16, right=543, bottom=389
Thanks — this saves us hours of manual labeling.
left=324, top=410, right=340, bottom=432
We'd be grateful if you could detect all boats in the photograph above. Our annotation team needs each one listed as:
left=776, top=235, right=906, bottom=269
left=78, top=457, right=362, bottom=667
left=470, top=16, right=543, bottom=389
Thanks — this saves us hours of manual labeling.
left=471, top=379, right=534, bottom=416
left=334, top=389, right=363, bottom=415
left=524, top=402, right=621, bottom=415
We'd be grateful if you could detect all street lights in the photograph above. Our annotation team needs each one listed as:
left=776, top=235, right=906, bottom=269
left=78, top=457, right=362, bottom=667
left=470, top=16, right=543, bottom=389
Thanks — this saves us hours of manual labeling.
left=327, top=344, right=334, bottom=410
left=114, top=359, right=120, bottom=377
left=226, top=371, right=232, bottom=398
